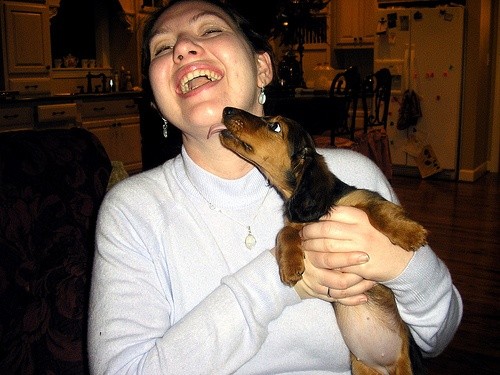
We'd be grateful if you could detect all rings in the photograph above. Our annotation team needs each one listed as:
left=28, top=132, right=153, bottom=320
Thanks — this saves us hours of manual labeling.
left=326, top=287, right=331, bottom=297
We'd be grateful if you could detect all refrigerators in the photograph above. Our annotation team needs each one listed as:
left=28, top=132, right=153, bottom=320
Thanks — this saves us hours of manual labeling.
left=373, top=6, right=464, bottom=181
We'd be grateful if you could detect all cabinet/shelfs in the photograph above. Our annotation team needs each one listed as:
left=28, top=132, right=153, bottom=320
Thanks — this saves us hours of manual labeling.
left=0, top=0, right=168, bottom=172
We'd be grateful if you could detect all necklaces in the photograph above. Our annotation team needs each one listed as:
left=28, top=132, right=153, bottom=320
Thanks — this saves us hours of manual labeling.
left=180, top=149, right=274, bottom=251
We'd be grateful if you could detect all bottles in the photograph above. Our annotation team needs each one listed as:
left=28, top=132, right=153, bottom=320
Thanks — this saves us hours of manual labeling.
left=312, top=62, right=336, bottom=90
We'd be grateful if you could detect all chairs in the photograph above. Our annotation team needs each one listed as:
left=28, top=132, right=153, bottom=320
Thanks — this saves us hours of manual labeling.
left=312, top=63, right=393, bottom=184
left=0, top=128, right=113, bottom=375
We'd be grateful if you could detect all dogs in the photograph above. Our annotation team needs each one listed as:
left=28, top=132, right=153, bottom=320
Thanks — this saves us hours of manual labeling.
left=206, top=106, right=432, bottom=375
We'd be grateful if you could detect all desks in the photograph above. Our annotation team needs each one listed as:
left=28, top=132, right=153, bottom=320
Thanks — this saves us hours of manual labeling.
left=292, top=93, right=350, bottom=139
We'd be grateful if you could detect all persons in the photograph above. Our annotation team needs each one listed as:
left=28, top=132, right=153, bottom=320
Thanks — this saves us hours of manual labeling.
left=86, top=1, right=466, bottom=374
